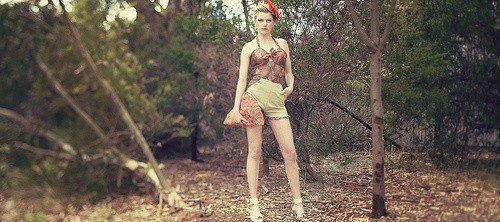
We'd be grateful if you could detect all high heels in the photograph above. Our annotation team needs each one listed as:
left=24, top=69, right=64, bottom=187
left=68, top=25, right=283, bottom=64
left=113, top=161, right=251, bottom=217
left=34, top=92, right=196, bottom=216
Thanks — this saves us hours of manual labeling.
left=247, top=203, right=263, bottom=222
left=291, top=203, right=310, bottom=222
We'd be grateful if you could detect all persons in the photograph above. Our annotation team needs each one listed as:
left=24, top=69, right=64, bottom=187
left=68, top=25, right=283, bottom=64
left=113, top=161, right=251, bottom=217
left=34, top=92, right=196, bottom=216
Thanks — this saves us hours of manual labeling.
left=223, top=0, right=312, bottom=222
